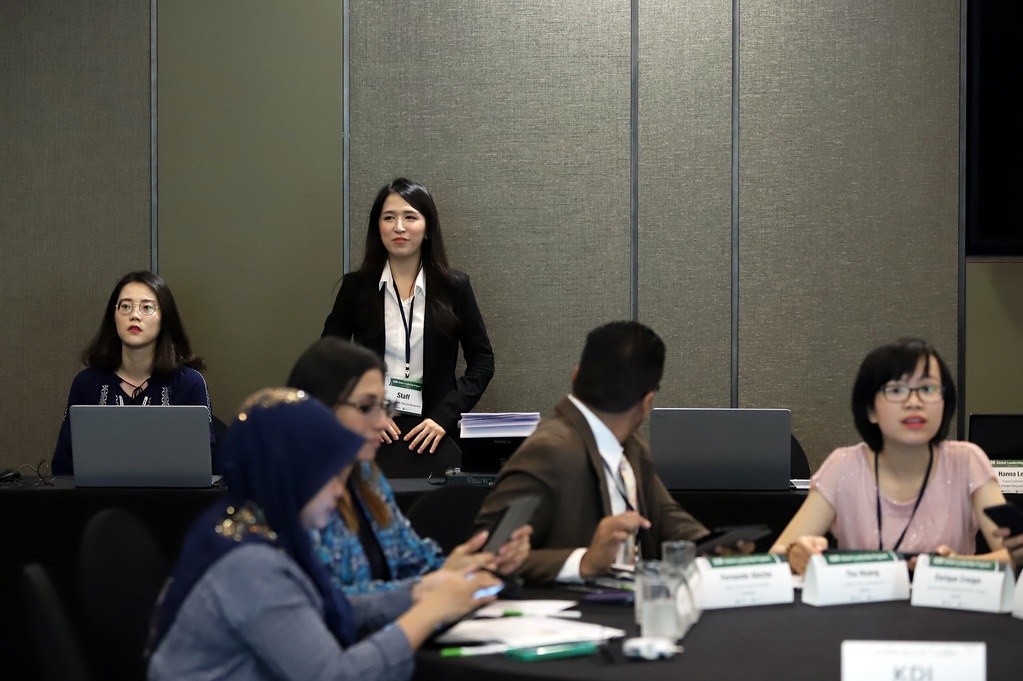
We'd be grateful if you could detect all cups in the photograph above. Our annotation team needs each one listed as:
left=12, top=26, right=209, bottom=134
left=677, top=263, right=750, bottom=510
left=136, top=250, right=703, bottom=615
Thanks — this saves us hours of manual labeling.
left=640, top=572, right=695, bottom=646
left=662, top=540, right=700, bottom=578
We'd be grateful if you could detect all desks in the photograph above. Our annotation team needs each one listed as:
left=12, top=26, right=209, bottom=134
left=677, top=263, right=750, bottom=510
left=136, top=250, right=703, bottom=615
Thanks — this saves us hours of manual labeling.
left=0, top=478, right=1023, bottom=681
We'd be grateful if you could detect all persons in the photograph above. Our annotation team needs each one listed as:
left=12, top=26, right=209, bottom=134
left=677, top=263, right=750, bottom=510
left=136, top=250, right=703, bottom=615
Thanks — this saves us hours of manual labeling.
left=136, top=335, right=535, bottom=681
left=321, top=178, right=494, bottom=477
left=49, top=268, right=217, bottom=476
left=472, top=318, right=755, bottom=584
left=765, top=336, right=1015, bottom=577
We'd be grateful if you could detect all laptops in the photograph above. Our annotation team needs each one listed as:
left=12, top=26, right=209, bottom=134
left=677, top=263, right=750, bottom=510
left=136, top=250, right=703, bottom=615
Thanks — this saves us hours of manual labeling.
left=649, top=407, right=792, bottom=493
left=70, top=404, right=222, bottom=489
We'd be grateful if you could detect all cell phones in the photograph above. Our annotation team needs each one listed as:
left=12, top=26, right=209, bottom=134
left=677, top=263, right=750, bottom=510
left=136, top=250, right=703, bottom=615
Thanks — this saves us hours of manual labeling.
left=478, top=495, right=543, bottom=555
left=465, top=571, right=505, bottom=599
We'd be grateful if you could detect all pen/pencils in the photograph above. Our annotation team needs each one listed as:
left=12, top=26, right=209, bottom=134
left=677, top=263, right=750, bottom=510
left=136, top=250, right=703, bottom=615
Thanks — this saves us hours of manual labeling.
left=560, top=585, right=604, bottom=594
left=502, top=608, right=581, bottom=619
left=440, top=644, right=510, bottom=660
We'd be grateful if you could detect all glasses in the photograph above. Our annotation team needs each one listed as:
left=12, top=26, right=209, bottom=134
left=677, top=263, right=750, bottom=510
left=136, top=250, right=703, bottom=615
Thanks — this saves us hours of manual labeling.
left=339, top=392, right=395, bottom=418
left=877, top=382, right=945, bottom=405
left=115, top=298, right=159, bottom=316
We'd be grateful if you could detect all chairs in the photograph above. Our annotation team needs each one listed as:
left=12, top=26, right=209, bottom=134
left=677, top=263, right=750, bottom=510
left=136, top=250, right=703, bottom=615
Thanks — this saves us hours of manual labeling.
left=405, top=478, right=495, bottom=550
left=967, top=412, right=1023, bottom=555
left=80, top=510, right=166, bottom=646
left=17, top=551, right=151, bottom=680
